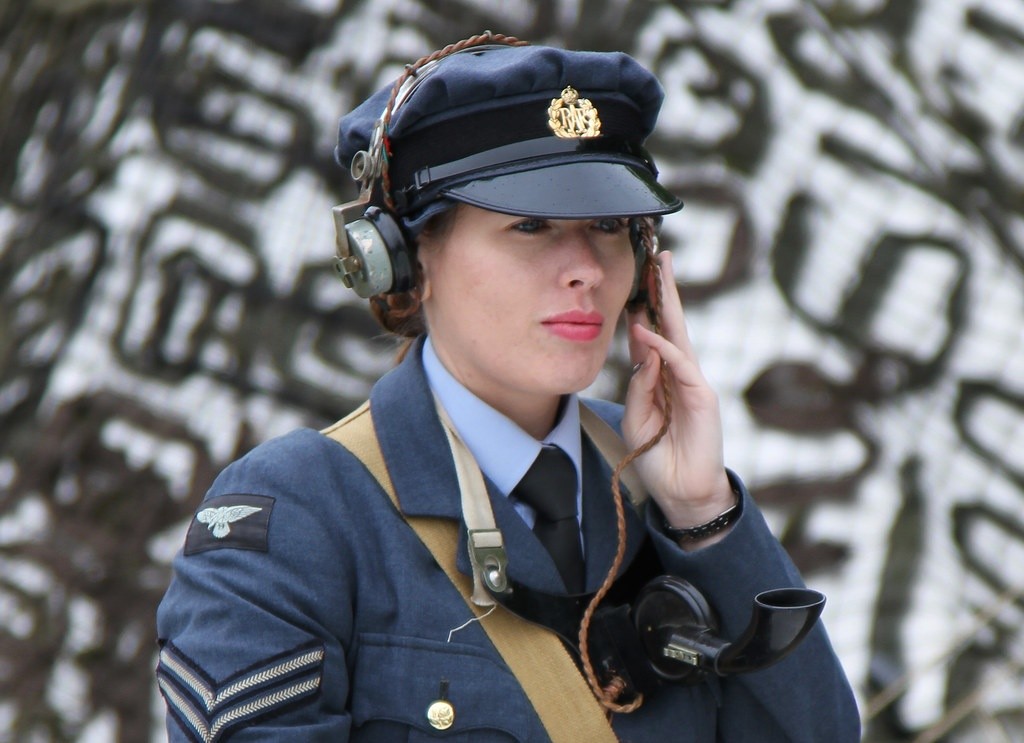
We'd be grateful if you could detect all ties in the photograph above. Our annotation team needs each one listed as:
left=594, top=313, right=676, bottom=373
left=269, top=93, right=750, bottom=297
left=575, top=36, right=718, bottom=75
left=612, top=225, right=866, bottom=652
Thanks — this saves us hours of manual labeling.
left=511, top=444, right=588, bottom=599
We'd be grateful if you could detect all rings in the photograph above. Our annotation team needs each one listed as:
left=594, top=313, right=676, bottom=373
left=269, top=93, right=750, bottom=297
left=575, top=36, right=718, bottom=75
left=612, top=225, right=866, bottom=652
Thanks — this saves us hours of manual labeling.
left=631, top=358, right=644, bottom=374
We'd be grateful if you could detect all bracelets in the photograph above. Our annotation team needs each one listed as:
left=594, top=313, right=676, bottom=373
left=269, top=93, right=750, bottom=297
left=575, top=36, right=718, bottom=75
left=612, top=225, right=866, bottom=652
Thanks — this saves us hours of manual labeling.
left=660, top=491, right=745, bottom=549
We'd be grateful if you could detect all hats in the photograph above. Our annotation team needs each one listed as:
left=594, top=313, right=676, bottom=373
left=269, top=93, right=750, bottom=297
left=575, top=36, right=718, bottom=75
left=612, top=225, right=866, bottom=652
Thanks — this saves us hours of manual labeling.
left=332, top=41, right=685, bottom=221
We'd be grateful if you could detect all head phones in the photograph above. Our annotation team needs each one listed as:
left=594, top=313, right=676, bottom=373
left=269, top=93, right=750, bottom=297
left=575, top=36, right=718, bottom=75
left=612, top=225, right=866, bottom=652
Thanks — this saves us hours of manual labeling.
left=330, top=29, right=660, bottom=311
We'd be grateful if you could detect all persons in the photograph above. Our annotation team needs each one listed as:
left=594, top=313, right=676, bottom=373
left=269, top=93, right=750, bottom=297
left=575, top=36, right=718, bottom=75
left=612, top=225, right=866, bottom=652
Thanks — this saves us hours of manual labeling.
left=154, top=32, right=864, bottom=743
